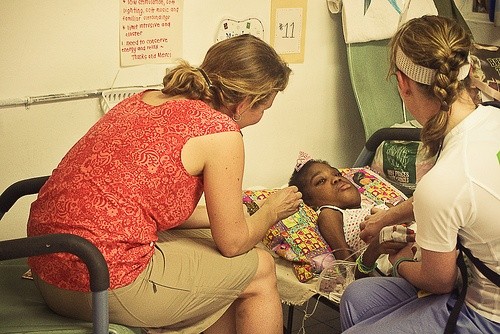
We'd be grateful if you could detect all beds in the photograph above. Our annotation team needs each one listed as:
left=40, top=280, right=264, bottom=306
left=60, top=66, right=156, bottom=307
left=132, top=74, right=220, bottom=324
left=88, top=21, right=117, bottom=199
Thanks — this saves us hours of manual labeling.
left=242, top=128, right=424, bottom=334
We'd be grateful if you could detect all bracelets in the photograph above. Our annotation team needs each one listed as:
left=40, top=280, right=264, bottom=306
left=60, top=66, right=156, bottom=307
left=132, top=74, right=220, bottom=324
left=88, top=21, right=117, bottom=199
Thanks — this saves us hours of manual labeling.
left=392, top=257, right=418, bottom=277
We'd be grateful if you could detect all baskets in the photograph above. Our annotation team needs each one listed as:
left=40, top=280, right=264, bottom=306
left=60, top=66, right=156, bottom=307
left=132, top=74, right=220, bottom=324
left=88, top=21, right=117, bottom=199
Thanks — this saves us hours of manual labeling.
left=100, top=88, right=147, bottom=114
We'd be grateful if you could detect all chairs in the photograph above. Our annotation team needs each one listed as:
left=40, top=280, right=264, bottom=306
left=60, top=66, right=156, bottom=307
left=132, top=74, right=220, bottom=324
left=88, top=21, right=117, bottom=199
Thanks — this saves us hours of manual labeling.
left=327, top=0, right=500, bottom=171
left=0, top=175, right=142, bottom=334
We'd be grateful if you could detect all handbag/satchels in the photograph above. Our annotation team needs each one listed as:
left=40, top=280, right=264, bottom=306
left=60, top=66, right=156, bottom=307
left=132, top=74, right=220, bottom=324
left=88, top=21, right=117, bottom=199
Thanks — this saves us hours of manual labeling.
left=370, top=119, right=439, bottom=190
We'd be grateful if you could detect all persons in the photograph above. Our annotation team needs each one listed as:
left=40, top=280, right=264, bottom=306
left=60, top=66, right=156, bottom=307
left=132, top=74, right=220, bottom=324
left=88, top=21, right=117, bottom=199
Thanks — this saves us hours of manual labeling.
left=289, top=159, right=421, bottom=281
left=339, top=14, right=500, bottom=334
left=27, top=34, right=303, bottom=334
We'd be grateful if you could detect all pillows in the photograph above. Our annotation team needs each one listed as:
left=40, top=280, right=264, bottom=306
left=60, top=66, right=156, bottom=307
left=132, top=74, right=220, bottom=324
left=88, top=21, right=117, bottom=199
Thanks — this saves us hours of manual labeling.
left=241, top=168, right=410, bottom=283
